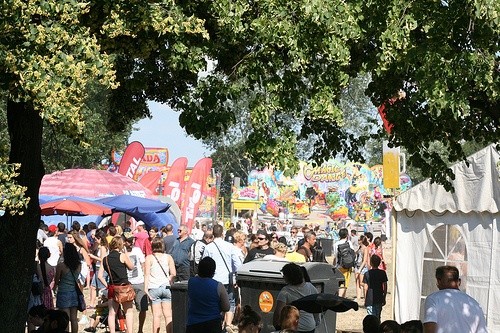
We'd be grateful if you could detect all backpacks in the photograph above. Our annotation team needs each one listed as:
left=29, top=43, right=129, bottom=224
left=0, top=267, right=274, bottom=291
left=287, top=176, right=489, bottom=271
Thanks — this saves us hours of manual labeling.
left=338, top=237, right=356, bottom=270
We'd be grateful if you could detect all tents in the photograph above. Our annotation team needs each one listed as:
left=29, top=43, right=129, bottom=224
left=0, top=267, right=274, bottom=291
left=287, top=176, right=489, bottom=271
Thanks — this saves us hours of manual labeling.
left=393, top=141, right=500, bottom=333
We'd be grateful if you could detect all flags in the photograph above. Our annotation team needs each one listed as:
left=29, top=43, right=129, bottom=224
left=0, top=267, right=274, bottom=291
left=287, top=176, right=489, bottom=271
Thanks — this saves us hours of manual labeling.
left=119, top=141, right=212, bottom=232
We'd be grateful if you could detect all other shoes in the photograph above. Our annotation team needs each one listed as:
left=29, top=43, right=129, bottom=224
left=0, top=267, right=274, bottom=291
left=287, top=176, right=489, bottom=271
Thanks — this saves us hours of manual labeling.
left=87, top=305, right=96, bottom=309
left=89, top=314, right=96, bottom=320
left=84, top=327, right=96, bottom=333
left=225, top=324, right=234, bottom=333
left=354, top=298, right=360, bottom=303
left=78, top=315, right=89, bottom=325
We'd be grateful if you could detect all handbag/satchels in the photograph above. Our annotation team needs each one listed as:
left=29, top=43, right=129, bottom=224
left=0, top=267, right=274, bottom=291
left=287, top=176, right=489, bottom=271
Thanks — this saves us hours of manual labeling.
left=114, top=284, right=135, bottom=303
left=229, top=272, right=238, bottom=290
left=76, top=284, right=86, bottom=312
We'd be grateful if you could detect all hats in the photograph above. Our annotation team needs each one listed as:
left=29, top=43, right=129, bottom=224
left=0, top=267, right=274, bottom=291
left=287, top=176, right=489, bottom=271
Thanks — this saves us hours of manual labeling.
left=71, top=223, right=80, bottom=230
left=286, top=237, right=298, bottom=252
left=122, top=232, right=135, bottom=242
left=49, top=225, right=59, bottom=232
left=136, top=220, right=145, bottom=227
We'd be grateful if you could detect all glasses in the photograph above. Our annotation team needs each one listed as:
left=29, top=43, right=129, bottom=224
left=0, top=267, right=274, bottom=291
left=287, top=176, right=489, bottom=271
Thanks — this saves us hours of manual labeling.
left=178, top=229, right=183, bottom=233
left=258, top=237, right=265, bottom=240
left=290, top=231, right=297, bottom=234
left=272, top=237, right=277, bottom=239
left=358, top=239, right=361, bottom=241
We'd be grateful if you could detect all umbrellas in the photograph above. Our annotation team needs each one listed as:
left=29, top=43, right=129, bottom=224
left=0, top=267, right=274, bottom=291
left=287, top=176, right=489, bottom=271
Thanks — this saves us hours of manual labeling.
left=39, top=198, right=112, bottom=231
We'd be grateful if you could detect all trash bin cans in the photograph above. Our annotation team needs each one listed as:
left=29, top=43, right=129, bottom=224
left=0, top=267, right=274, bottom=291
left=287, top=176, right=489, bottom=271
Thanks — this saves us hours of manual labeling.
left=235, top=256, right=345, bottom=333
left=320, top=238, right=334, bottom=256
left=166, top=282, right=189, bottom=333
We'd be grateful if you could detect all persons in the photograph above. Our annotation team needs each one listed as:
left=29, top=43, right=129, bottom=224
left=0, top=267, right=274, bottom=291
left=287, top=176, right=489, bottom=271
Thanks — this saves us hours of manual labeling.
left=236, top=262, right=319, bottom=333
left=187, top=256, right=230, bottom=333
left=423, top=266, right=488, bottom=333
left=187, top=231, right=214, bottom=278
left=225, top=218, right=321, bottom=263
left=27, top=221, right=194, bottom=333
left=325, top=220, right=386, bottom=305
left=202, top=224, right=242, bottom=333
left=362, top=315, right=423, bottom=333
left=362, top=254, right=387, bottom=321
